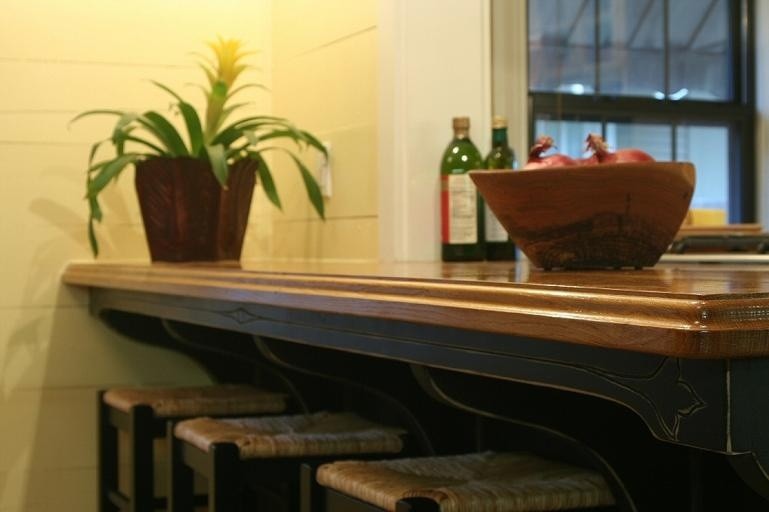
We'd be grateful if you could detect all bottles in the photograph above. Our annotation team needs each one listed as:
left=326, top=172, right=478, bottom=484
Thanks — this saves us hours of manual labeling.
left=438, top=115, right=487, bottom=262
left=479, top=115, right=517, bottom=263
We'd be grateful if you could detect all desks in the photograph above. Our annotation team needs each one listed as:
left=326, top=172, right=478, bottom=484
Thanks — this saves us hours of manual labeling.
left=59, top=261, right=769, bottom=512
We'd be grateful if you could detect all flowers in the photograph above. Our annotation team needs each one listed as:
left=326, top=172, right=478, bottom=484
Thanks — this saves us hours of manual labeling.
left=70, top=30, right=330, bottom=255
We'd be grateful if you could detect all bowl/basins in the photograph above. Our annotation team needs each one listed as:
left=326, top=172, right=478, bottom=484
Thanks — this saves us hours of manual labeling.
left=467, top=161, right=697, bottom=272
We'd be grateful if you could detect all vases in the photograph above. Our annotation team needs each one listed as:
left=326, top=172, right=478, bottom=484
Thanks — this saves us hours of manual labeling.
left=134, top=159, right=260, bottom=267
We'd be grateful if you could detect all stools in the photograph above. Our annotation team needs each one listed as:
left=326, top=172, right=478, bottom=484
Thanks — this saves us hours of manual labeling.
left=170, top=412, right=405, bottom=511
left=96, top=386, right=297, bottom=510
left=300, top=448, right=615, bottom=510
